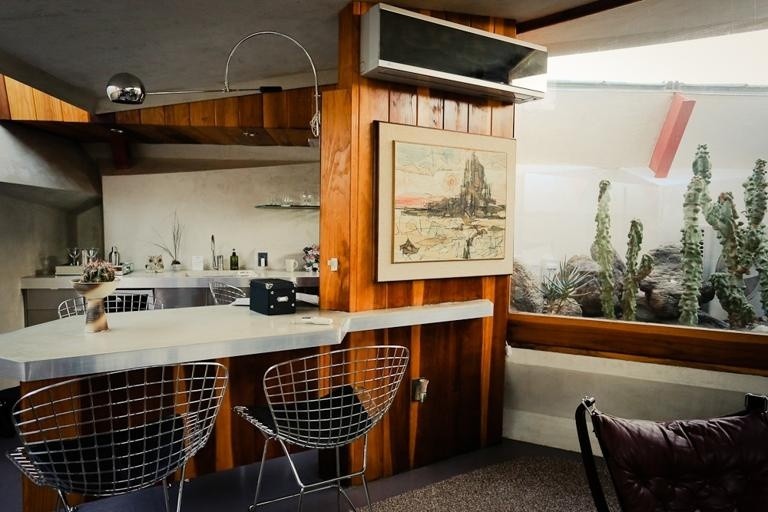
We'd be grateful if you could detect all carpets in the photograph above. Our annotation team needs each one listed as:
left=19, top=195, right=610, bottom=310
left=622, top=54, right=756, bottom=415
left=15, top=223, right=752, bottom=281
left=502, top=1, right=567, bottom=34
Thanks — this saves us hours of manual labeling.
left=349, top=450, right=625, bottom=512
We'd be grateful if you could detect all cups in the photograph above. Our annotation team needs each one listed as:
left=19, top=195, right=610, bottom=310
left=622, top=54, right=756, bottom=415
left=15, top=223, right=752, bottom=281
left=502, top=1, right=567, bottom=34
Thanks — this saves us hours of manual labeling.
left=286, top=259, right=298, bottom=273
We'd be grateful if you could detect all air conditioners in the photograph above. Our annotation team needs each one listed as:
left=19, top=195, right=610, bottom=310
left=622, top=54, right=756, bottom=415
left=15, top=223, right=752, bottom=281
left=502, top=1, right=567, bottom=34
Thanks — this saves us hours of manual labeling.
left=359, top=3, right=548, bottom=105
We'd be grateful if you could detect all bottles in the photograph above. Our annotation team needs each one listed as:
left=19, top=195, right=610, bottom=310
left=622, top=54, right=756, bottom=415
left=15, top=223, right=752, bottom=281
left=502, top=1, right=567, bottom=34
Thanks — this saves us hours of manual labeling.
left=108, top=246, right=119, bottom=265
left=230, top=249, right=238, bottom=270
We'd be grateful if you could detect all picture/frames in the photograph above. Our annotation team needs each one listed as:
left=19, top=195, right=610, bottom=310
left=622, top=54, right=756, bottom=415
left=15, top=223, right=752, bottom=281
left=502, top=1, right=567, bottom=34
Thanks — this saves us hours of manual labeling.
left=369, top=119, right=518, bottom=285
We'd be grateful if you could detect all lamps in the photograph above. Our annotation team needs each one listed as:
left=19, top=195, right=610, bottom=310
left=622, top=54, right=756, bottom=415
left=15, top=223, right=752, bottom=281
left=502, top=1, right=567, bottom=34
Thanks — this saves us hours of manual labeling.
left=105, top=32, right=321, bottom=139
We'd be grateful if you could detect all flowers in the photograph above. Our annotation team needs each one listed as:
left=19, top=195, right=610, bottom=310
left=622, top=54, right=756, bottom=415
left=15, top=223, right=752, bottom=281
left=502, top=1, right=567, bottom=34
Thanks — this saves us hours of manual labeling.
left=303, top=243, right=320, bottom=274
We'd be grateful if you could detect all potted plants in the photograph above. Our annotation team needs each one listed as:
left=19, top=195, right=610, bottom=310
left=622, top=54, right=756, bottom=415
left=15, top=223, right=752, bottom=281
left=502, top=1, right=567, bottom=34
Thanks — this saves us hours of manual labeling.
left=68, top=258, right=120, bottom=334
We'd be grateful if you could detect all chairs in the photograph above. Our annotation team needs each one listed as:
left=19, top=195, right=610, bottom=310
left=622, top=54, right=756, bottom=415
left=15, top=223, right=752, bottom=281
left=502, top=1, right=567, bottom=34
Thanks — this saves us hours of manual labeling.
left=232, top=343, right=411, bottom=512
left=57, top=292, right=164, bottom=318
left=208, top=280, right=246, bottom=306
left=576, top=391, right=767, bottom=512
left=4, top=361, right=230, bottom=512
